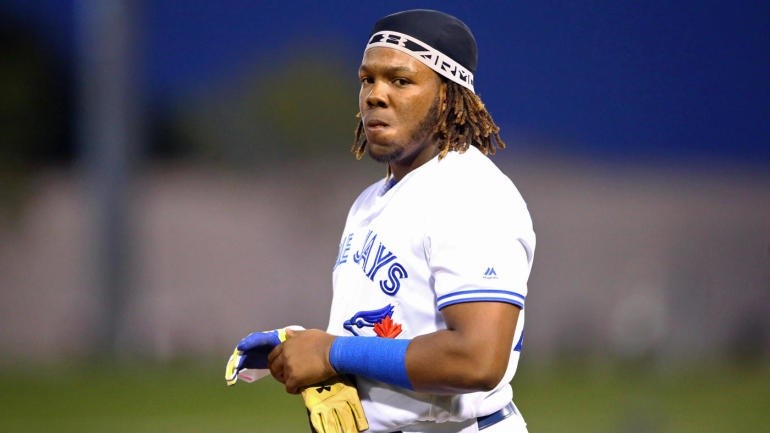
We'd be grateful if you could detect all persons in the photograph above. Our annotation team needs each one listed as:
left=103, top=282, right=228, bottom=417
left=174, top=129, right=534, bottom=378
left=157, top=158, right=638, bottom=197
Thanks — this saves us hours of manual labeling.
left=226, top=8, right=537, bottom=433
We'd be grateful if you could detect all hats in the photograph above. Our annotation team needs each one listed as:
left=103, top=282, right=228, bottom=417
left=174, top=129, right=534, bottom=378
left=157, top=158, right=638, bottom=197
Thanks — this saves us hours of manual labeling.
left=363, top=8, right=478, bottom=92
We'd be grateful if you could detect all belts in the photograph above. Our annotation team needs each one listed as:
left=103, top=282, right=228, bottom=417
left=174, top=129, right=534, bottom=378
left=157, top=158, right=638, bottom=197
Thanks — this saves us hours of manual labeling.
left=388, top=403, right=514, bottom=433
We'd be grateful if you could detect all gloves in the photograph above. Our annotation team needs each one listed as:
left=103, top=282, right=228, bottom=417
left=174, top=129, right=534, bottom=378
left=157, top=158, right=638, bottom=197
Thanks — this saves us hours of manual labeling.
left=299, top=373, right=367, bottom=433
left=224, top=325, right=306, bottom=385
